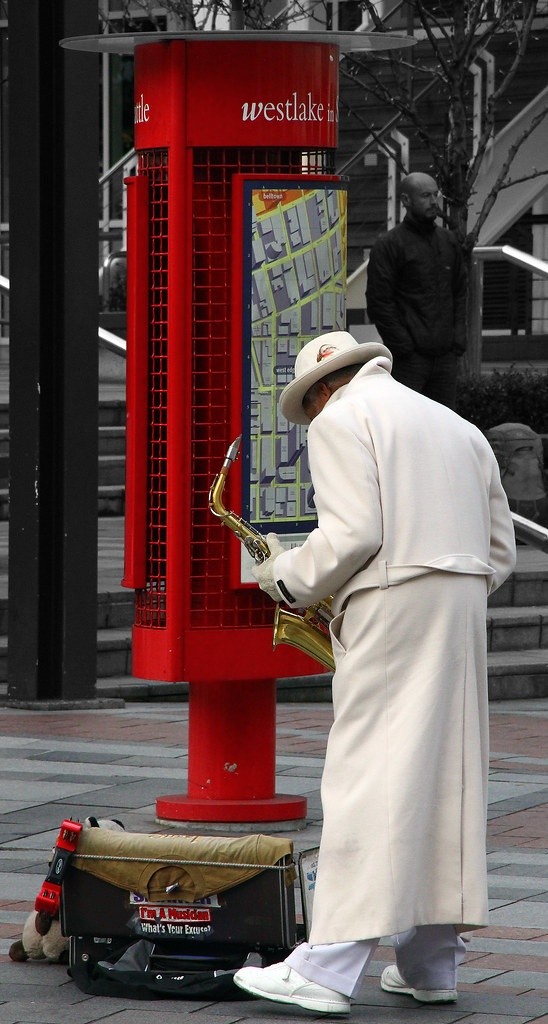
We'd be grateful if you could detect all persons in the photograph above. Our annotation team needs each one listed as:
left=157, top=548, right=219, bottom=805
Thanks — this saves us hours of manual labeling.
left=362, top=170, right=474, bottom=414
left=230, top=332, right=518, bottom=1011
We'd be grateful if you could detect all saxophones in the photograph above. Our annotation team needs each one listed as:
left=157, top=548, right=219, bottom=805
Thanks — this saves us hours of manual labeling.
left=206, top=434, right=334, bottom=670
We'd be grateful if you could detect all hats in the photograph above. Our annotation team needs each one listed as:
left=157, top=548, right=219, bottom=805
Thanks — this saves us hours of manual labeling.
left=280, top=330, right=393, bottom=426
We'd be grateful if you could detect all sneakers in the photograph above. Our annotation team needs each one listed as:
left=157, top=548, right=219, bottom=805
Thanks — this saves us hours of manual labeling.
left=381, top=965, right=457, bottom=1003
left=234, top=964, right=351, bottom=1015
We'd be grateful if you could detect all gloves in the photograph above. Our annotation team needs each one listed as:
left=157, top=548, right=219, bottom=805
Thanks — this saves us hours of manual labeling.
left=251, top=532, right=285, bottom=603
